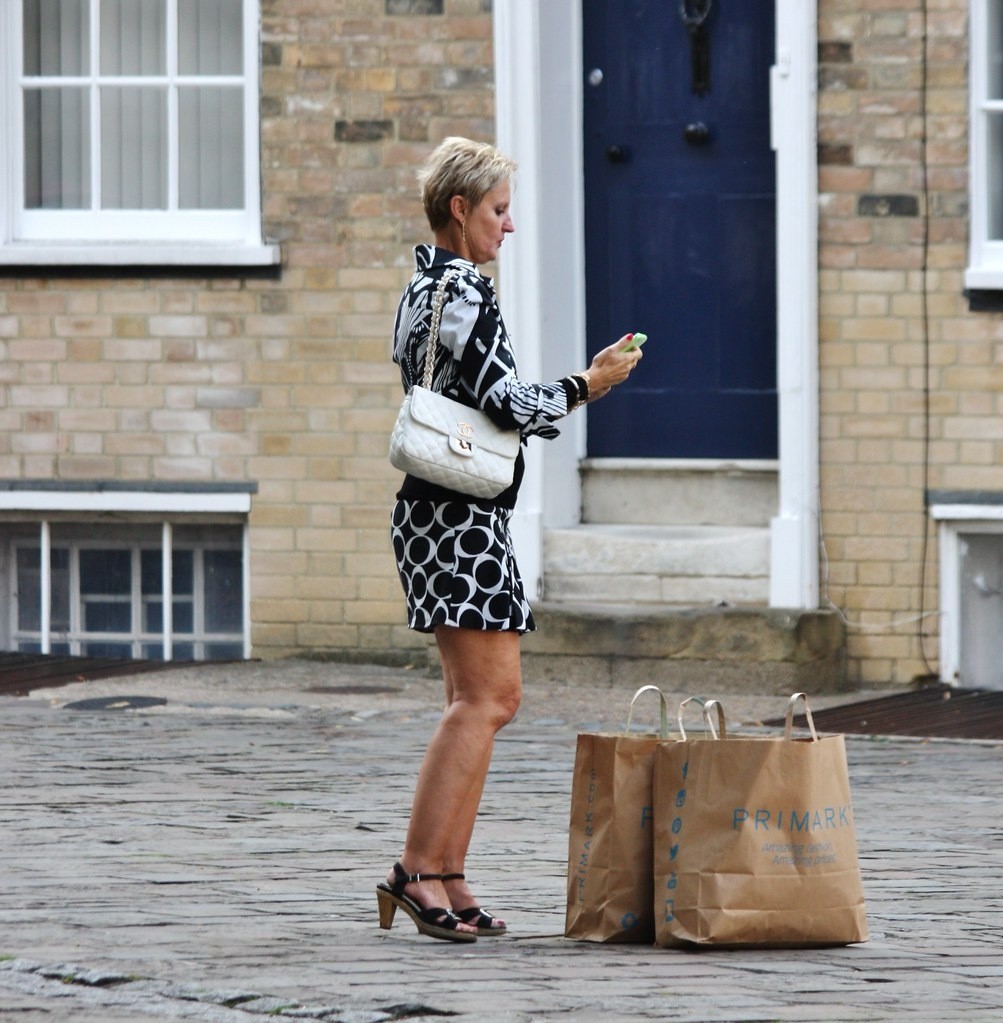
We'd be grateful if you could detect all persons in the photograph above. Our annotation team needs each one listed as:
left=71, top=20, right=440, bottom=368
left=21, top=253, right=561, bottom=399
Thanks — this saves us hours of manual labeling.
left=373, top=137, right=643, bottom=943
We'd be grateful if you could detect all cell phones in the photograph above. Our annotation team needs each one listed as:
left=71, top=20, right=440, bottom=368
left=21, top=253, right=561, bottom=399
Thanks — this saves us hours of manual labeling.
left=620, top=333, right=647, bottom=354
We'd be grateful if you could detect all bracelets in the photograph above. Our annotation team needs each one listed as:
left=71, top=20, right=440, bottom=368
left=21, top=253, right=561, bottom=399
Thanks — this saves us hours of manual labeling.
left=565, top=372, right=590, bottom=414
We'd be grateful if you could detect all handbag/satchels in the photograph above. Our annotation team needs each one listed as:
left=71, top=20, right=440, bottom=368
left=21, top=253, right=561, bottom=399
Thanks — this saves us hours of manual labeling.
left=390, top=267, right=521, bottom=497
left=651, top=692, right=871, bottom=946
left=563, top=685, right=725, bottom=943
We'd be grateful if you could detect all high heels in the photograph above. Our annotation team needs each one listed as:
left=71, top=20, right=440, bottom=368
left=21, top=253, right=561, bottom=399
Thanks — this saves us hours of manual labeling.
left=376, top=861, right=479, bottom=942
left=442, top=871, right=507, bottom=935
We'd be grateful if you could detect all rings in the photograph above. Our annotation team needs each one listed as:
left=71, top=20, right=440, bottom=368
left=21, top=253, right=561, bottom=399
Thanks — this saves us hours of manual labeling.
left=634, top=362, right=636, bottom=369
